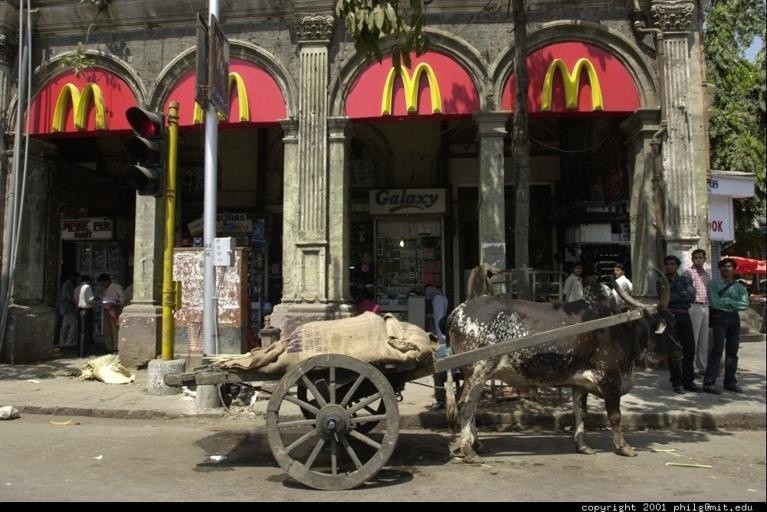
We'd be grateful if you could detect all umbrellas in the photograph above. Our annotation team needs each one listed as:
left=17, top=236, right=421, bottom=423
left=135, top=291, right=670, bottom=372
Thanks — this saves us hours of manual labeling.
left=737, top=262, right=767, bottom=294
left=721, top=254, right=767, bottom=273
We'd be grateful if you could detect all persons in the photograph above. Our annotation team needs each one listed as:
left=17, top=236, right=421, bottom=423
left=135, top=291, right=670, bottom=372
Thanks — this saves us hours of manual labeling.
left=703, top=257, right=751, bottom=396
left=96, top=273, right=127, bottom=355
left=585, top=279, right=611, bottom=310
left=683, top=249, right=722, bottom=380
left=429, top=334, right=452, bottom=413
left=560, top=261, right=585, bottom=304
left=422, top=282, right=449, bottom=344
left=610, top=261, right=634, bottom=308
left=72, top=273, right=100, bottom=358
left=57, top=268, right=82, bottom=347
left=656, top=254, right=704, bottom=395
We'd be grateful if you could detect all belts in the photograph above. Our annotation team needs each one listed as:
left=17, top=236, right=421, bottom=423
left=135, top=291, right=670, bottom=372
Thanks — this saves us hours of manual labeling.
left=695, top=302, right=708, bottom=304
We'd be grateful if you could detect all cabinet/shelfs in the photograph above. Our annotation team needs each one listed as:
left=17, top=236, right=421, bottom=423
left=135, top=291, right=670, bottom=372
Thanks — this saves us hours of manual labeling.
left=350, top=214, right=446, bottom=305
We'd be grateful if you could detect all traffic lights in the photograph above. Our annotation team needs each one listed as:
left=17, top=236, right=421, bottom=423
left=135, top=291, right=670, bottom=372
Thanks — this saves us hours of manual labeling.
left=125, top=103, right=167, bottom=199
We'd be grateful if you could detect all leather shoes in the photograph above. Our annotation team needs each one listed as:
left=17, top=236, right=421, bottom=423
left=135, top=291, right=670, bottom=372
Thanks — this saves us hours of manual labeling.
left=672, top=382, right=744, bottom=394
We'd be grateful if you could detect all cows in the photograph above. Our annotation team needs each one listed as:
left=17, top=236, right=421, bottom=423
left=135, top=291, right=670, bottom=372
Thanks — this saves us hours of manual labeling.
left=443, top=266, right=684, bottom=465
